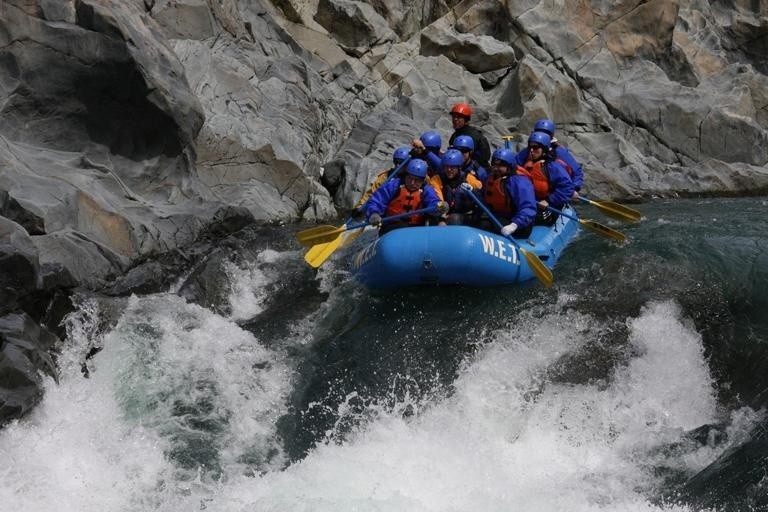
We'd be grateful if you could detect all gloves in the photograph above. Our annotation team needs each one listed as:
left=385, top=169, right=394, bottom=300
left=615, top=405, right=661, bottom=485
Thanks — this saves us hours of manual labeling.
left=460, top=181, right=473, bottom=194
left=368, top=213, right=382, bottom=226
left=501, top=222, right=518, bottom=236
left=439, top=201, right=448, bottom=213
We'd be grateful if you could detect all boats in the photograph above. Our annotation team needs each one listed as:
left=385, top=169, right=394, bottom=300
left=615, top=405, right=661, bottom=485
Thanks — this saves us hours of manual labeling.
left=344, top=202, right=582, bottom=297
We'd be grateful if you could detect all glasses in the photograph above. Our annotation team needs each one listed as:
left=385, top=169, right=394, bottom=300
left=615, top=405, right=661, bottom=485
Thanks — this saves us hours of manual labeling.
left=527, top=145, right=539, bottom=150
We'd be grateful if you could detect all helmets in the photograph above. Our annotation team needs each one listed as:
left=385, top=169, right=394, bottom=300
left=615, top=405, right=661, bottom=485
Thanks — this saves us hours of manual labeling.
left=421, top=130, right=441, bottom=148
left=394, top=148, right=410, bottom=159
left=453, top=135, right=474, bottom=151
left=441, top=149, right=463, bottom=167
left=449, top=103, right=472, bottom=121
left=407, top=158, right=428, bottom=178
left=528, top=131, right=550, bottom=148
left=494, top=148, right=517, bottom=166
left=535, top=119, right=554, bottom=134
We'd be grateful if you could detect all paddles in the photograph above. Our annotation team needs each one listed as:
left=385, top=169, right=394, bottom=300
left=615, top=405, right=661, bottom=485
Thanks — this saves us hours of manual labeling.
left=536, top=202, right=625, bottom=245
left=577, top=196, right=641, bottom=224
left=466, top=189, right=553, bottom=289
left=298, top=153, right=441, bottom=269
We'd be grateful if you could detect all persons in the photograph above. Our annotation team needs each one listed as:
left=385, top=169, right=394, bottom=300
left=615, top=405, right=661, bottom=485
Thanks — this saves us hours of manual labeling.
left=352, top=104, right=583, bottom=239
